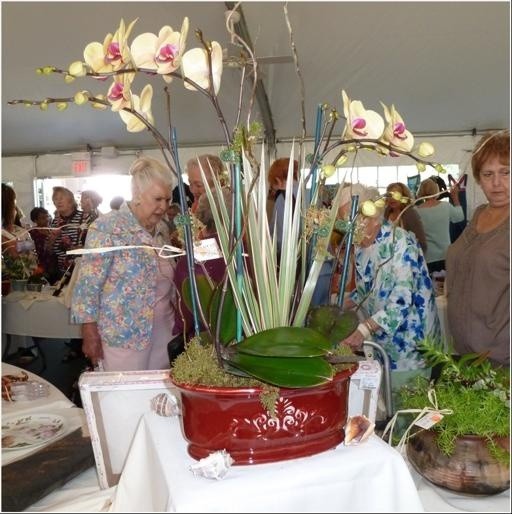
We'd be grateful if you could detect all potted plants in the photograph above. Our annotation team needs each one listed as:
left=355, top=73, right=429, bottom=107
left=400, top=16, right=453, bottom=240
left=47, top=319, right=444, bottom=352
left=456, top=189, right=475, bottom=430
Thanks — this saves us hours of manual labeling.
left=393, top=332, right=511, bottom=495
left=6, top=255, right=35, bottom=291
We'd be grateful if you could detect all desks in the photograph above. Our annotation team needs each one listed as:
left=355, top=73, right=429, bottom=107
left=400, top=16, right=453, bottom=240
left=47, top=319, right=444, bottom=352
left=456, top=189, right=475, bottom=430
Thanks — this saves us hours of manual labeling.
left=2, top=286, right=83, bottom=377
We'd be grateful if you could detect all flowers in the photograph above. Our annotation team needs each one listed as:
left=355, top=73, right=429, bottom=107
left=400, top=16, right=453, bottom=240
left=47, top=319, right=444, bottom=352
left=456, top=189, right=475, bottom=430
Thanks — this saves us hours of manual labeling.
left=28, top=267, right=49, bottom=285
left=7, top=1, right=511, bottom=419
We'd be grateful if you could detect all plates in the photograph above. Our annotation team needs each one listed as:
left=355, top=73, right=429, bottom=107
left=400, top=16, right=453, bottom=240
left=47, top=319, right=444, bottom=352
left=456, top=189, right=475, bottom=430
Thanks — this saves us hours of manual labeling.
left=2, top=414, right=64, bottom=451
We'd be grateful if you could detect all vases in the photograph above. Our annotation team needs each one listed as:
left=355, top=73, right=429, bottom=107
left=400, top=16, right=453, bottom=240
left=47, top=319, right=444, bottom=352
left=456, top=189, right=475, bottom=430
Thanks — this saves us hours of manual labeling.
left=169, top=361, right=360, bottom=466
left=27, top=283, right=42, bottom=292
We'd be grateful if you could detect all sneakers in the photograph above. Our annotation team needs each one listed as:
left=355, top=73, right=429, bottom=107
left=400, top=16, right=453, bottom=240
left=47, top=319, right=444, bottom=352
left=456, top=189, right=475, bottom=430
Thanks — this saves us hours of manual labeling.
left=6, top=344, right=76, bottom=366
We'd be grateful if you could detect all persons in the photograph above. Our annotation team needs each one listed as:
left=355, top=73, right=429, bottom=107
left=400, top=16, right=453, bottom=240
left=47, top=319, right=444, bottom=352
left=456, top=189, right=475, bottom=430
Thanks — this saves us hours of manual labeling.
left=446, top=129, right=511, bottom=368
